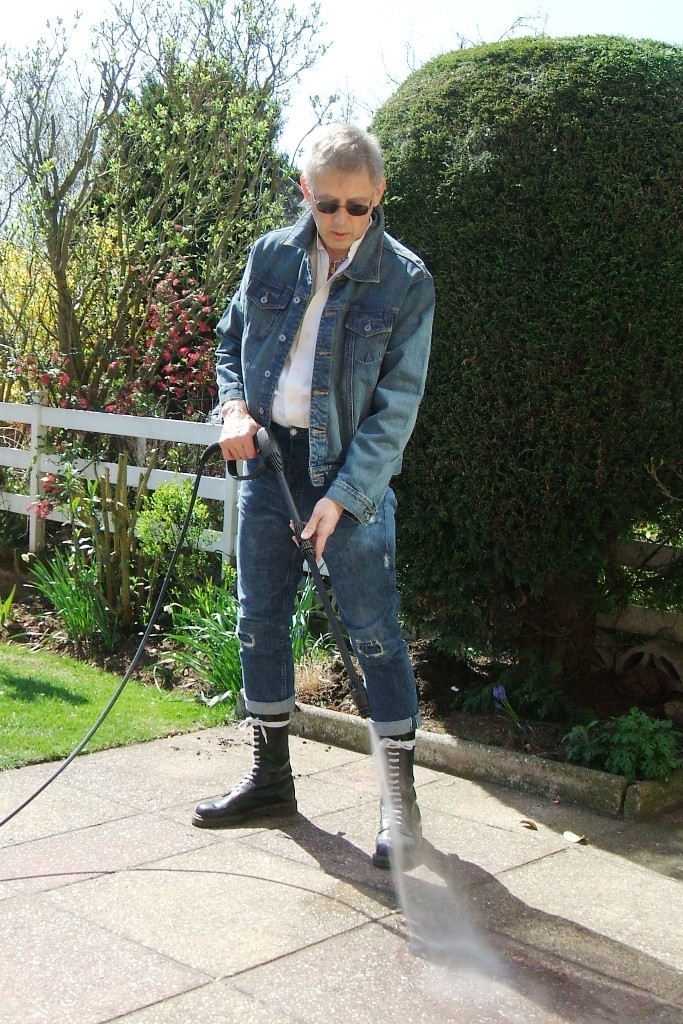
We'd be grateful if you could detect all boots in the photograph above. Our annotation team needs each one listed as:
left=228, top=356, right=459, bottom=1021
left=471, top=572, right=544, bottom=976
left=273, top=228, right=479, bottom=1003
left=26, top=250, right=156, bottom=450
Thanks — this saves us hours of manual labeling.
left=372, top=728, right=422, bottom=869
left=196, top=710, right=299, bottom=828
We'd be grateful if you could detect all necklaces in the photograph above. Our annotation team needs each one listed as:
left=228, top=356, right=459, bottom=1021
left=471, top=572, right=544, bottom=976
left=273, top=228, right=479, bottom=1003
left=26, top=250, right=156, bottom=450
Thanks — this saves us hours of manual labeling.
left=328, top=256, right=347, bottom=274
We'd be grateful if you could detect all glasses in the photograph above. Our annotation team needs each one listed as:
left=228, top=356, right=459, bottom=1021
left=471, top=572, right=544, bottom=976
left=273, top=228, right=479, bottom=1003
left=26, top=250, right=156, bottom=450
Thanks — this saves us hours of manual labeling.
left=310, top=185, right=374, bottom=216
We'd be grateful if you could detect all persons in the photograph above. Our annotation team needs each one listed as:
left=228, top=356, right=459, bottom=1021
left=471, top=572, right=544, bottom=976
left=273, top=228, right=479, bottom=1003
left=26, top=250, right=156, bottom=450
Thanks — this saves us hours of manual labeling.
left=192, top=123, right=436, bottom=871
